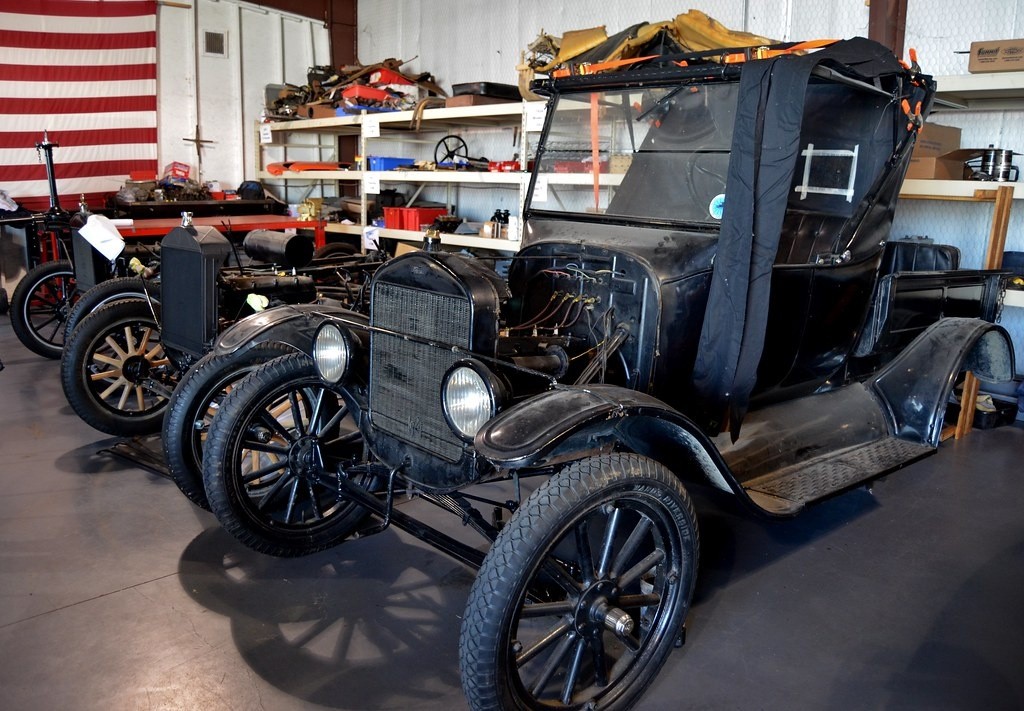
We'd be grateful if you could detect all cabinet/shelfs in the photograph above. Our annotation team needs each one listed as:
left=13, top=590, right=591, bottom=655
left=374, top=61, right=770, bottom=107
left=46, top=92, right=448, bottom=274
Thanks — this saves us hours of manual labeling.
left=256, top=73, right=1024, bottom=311
left=113, top=197, right=273, bottom=220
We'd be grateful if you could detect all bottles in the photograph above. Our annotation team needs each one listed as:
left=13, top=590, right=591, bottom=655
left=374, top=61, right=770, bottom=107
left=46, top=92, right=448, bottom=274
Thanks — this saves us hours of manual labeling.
left=988, top=144, right=994, bottom=149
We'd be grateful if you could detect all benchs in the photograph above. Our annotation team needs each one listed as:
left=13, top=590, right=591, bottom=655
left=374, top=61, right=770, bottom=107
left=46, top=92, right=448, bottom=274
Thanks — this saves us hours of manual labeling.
left=775, top=205, right=850, bottom=267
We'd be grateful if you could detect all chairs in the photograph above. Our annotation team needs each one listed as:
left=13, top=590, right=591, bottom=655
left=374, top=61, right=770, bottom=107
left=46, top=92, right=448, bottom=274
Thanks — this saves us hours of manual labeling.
left=876, top=241, right=960, bottom=353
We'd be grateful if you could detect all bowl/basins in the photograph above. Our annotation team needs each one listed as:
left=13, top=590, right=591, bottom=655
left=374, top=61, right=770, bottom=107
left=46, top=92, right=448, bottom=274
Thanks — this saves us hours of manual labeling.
left=288, top=203, right=306, bottom=218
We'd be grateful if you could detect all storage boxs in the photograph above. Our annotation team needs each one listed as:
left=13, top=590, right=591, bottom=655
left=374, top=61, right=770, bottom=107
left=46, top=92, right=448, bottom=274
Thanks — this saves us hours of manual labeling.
left=554, top=161, right=585, bottom=174
left=913, top=122, right=961, bottom=157
left=394, top=242, right=460, bottom=256
left=366, top=156, right=415, bottom=172
left=382, top=206, right=408, bottom=230
left=379, top=84, right=420, bottom=106
left=501, top=161, right=521, bottom=172
left=585, top=161, right=607, bottom=173
left=968, top=38, right=1024, bottom=73
left=400, top=207, right=448, bottom=231
left=342, top=85, right=391, bottom=102
left=905, top=147, right=989, bottom=181
left=370, top=69, right=413, bottom=85
left=947, top=393, right=1019, bottom=431
left=165, top=162, right=191, bottom=179
left=488, top=161, right=502, bottom=173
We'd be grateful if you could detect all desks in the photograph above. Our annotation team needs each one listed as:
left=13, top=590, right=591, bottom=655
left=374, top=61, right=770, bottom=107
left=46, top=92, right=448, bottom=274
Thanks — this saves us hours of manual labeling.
left=113, top=214, right=326, bottom=258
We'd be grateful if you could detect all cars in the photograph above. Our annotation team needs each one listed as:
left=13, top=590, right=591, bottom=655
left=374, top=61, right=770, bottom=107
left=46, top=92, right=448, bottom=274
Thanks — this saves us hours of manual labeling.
left=9, top=28, right=1018, bottom=711
